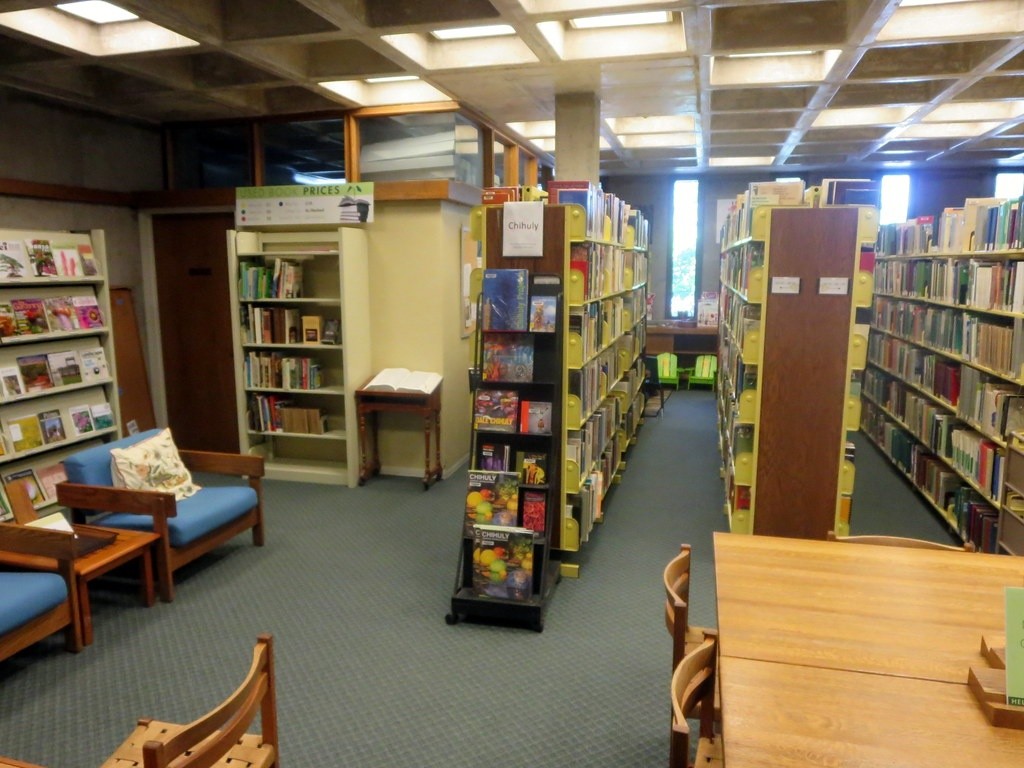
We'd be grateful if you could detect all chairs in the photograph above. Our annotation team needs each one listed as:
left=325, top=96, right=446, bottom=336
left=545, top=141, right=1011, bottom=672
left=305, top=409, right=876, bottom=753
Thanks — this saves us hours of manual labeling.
left=0, top=522, right=83, bottom=662
left=663, top=543, right=721, bottom=733
left=56, top=428, right=265, bottom=602
left=669, top=629, right=723, bottom=768
left=98, top=634, right=280, bottom=768
left=657, top=352, right=684, bottom=390
left=685, top=355, right=718, bottom=392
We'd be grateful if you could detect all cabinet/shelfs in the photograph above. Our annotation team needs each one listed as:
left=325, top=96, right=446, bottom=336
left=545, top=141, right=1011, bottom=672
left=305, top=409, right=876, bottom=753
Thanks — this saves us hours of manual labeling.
left=717, top=204, right=879, bottom=540
left=468, top=203, right=650, bottom=579
left=0, top=228, right=123, bottom=522
left=226, top=227, right=372, bottom=488
left=859, top=249, right=1024, bottom=547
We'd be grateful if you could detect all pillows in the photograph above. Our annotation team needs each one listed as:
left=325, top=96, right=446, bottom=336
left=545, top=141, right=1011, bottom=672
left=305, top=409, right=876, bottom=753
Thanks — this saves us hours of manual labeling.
left=109, top=428, right=200, bottom=502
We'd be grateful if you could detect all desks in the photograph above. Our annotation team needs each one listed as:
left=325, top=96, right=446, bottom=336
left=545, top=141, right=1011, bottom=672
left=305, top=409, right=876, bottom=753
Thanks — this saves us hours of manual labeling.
left=0, top=524, right=161, bottom=646
left=354, top=376, right=442, bottom=491
left=646, top=325, right=718, bottom=355
left=712, top=530, right=1024, bottom=768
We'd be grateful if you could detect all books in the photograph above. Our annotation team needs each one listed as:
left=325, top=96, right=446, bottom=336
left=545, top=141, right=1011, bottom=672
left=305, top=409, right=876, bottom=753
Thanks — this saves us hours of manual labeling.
left=457, top=177, right=1024, bottom=604
left=0, top=240, right=115, bottom=521
left=236, top=256, right=342, bottom=435
